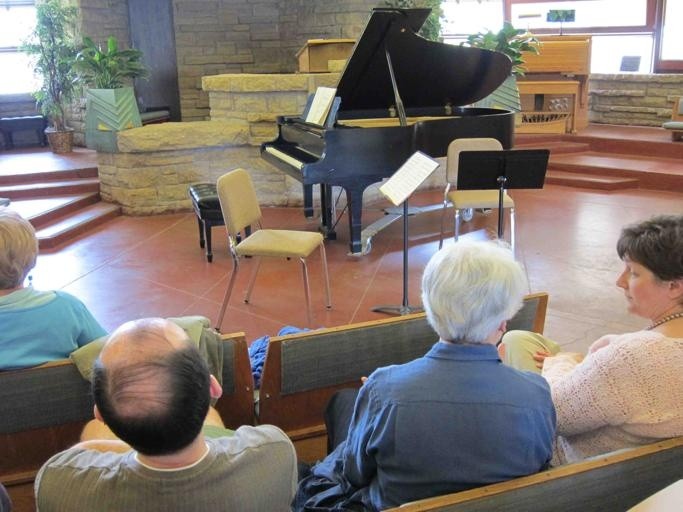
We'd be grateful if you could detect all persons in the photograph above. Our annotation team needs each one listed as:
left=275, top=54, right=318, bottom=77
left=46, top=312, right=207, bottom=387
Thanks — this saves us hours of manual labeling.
left=323, top=233, right=555, bottom=511
left=497, top=215, right=682, bottom=470
left=1, top=216, right=111, bottom=369
left=34, top=318, right=299, bottom=512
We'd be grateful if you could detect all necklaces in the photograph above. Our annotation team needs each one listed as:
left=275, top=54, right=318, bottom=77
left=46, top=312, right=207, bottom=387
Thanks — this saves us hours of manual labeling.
left=645, top=311, right=683, bottom=331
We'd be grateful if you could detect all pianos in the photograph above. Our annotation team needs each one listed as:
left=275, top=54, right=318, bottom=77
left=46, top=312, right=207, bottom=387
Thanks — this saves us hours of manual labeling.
left=260, top=7, right=516, bottom=254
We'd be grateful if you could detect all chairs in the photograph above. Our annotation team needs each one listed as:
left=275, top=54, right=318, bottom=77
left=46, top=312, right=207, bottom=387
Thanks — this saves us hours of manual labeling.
left=213, top=168, right=333, bottom=333
left=435, top=139, right=517, bottom=260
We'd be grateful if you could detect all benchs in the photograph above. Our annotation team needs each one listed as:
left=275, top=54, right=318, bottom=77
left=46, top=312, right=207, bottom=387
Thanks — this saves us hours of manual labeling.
left=0, top=292, right=683, bottom=512
left=1, top=115, right=48, bottom=149
left=515, top=79, right=580, bottom=134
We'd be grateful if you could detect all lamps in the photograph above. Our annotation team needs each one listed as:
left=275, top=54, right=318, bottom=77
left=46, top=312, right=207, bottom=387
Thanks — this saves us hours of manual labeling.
left=546, top=10, right=575, bottom=34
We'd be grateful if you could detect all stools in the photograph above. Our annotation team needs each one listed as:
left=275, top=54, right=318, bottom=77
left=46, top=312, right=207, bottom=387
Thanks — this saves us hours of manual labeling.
left=186, top=182, right=255, bottom=261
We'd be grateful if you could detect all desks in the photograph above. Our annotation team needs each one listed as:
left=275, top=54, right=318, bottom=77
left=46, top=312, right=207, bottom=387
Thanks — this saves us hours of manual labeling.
left=510, top=33, right=592, bottom=129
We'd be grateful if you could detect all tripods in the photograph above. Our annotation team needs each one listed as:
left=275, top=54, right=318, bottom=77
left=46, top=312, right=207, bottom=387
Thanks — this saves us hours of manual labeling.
left=370, top=202, right=428, bottom=320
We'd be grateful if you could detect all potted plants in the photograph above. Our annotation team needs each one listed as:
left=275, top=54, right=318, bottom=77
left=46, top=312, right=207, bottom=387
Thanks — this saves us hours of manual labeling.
left=25, top=1, right=152, bottom=154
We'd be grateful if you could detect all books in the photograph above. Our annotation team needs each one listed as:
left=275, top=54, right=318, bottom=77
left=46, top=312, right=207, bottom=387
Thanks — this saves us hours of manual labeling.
left=306, top=86, right=338, bottom=127
